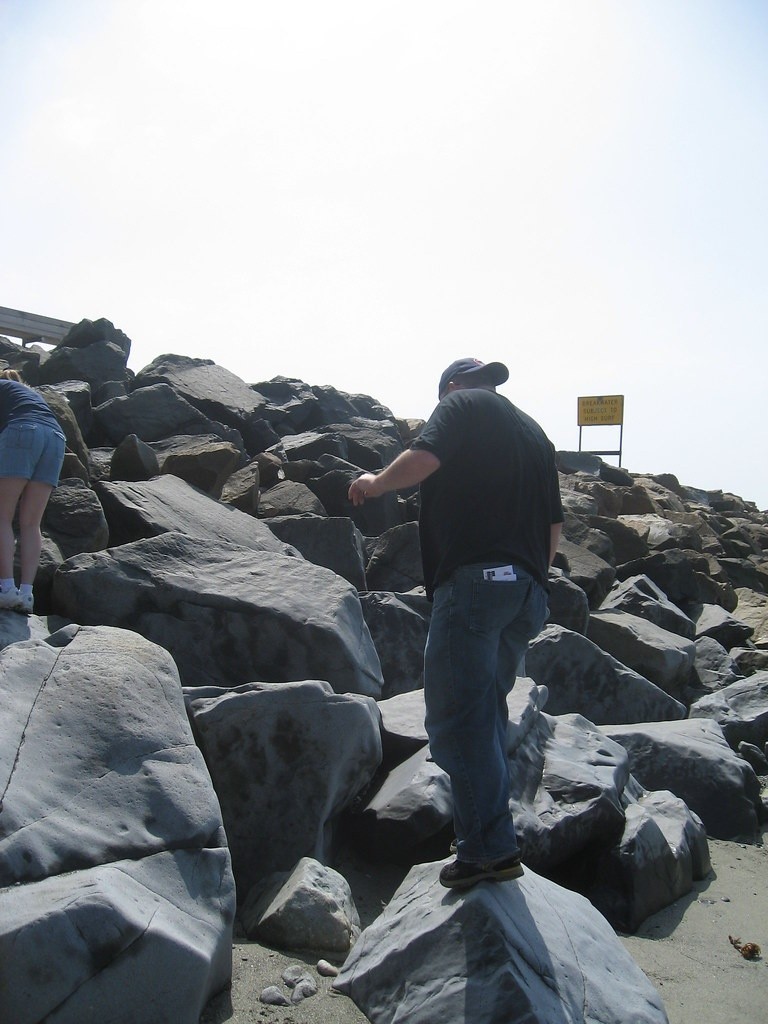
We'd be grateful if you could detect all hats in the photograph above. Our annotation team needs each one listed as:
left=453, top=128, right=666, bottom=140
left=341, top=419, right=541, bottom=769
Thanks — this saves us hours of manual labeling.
left=438, top=357, right=509, bottom=401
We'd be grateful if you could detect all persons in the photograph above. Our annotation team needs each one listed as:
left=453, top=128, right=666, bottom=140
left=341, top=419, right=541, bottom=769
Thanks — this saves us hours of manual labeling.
left=349, top=357, right=565, bottom=889
left=0, top=368, right=67, bottom=615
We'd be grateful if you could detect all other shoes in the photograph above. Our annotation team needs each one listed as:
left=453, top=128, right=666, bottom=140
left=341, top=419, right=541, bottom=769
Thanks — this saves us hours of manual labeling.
left=0, top=587, right=23, bottom=609
left=12, top=593, right=34, bottom=613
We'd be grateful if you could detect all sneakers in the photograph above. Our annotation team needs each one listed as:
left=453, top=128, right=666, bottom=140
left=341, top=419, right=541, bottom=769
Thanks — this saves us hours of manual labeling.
left=450, top=839, right=457, bottom=854
left=439, top=848, right=524, bottom=888
